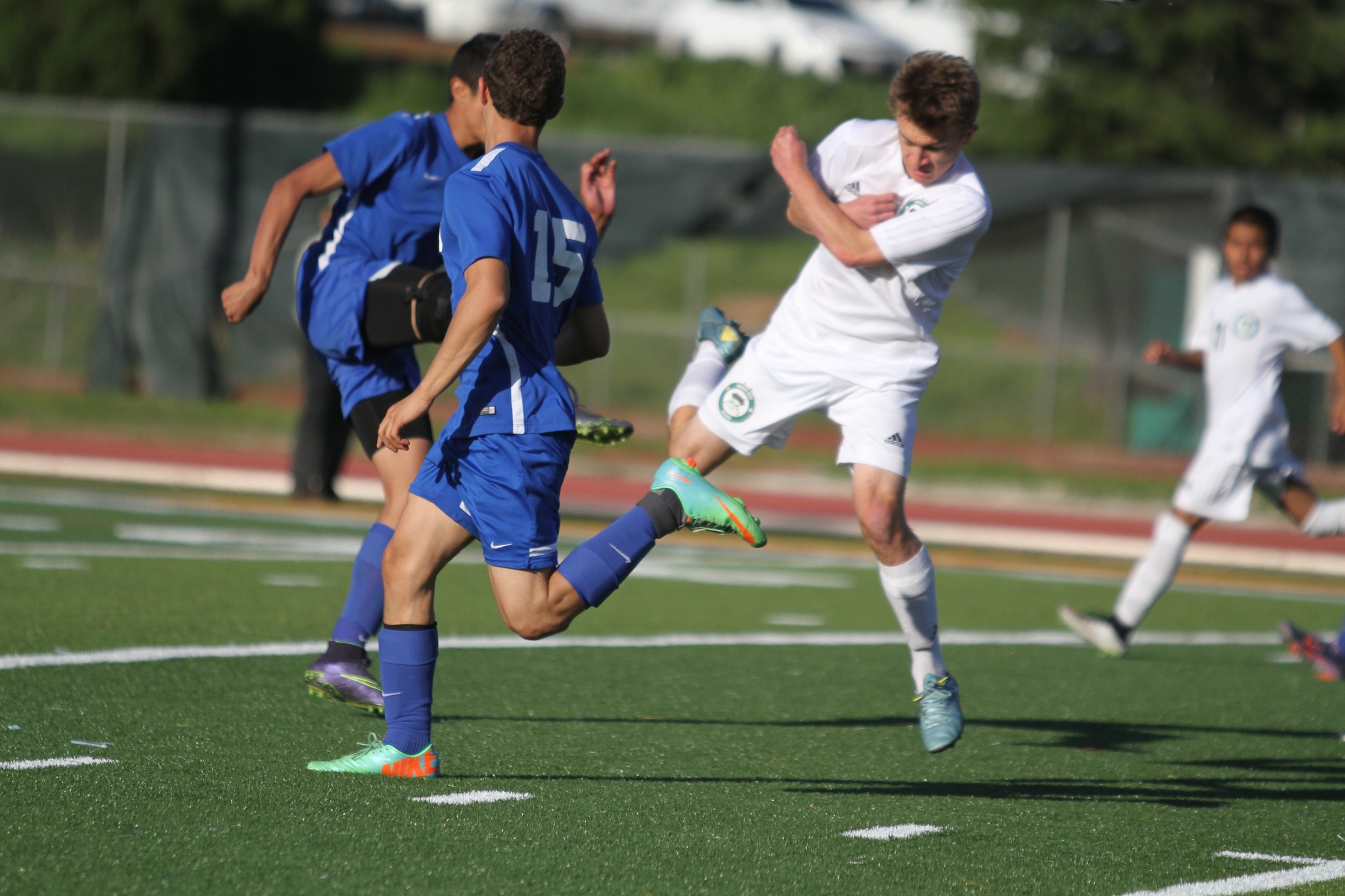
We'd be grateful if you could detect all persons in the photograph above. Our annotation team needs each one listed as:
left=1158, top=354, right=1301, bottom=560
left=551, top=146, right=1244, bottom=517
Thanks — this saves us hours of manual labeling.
left=307, top=30, right=767, bottom=780
left=666, top=53, right=990, bottom=754
left=1061, top=206, right=1345, bottom=659
left=221, top=32, right=632, bottom=719
left=1279, top=618, right=1345, bottom=680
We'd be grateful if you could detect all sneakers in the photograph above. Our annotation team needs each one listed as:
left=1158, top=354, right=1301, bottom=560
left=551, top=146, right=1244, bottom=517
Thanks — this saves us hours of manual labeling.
left=565, top=381, right=634, bottom=446
left=910, top=672, right=963, bottom=753
left=1058, top=606, right=1129, bottom=658
left=307, top=732, right=440, bottom=777
left=1281, top=620, right=1345, bottom=683
left=698, top=306, right=749, bottom=364
left=303, top=655, right=387, bottom=716
left=651, top=458, right=767, bottom=549
